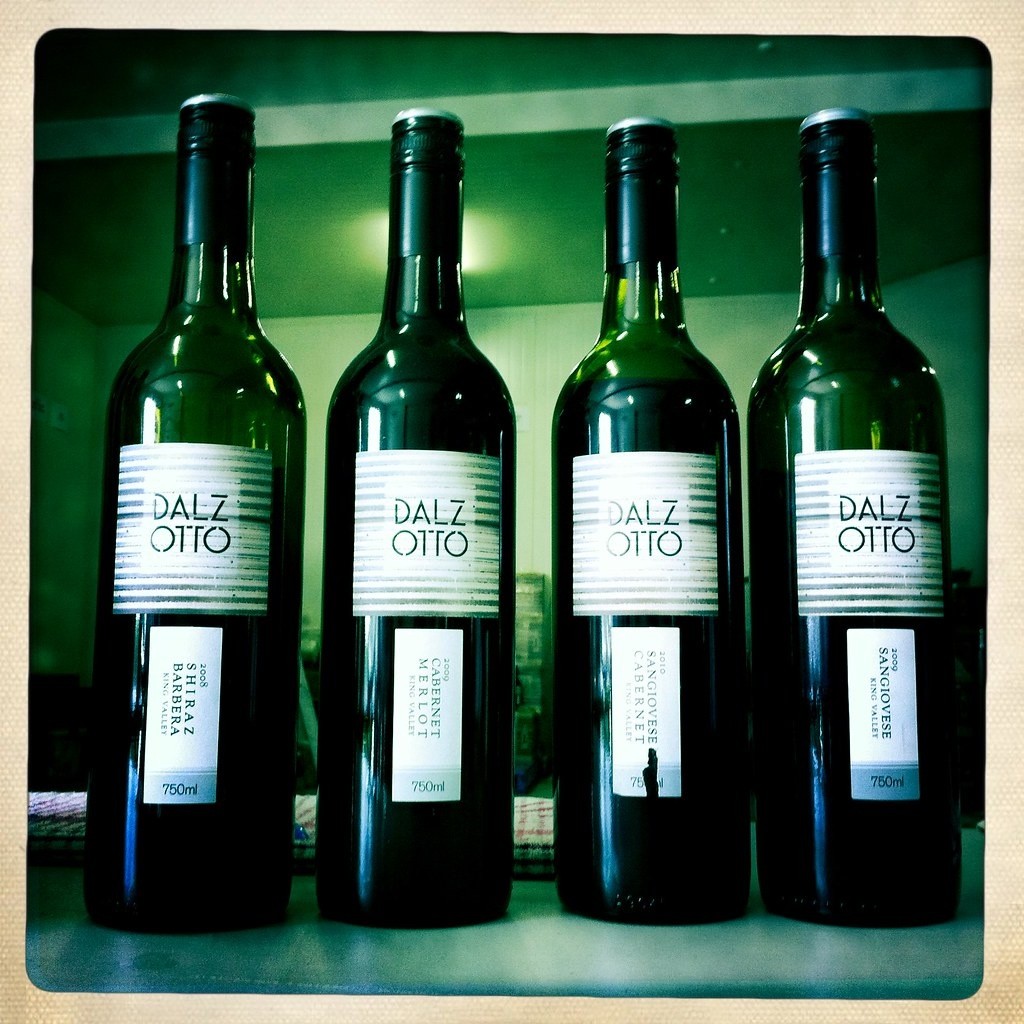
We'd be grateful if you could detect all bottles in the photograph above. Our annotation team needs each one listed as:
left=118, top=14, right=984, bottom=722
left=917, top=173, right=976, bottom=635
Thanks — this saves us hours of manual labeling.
left=551, top=115, right=752, bottom=927
left=746, top=106, right=961, bottom=930
left=83, top=93, right=305, bottom=936
left=292, top=654, right=320, bottom=883
left=514, top=564, right=552, bottom=884
left=314, top=106, right=518, bottom=930
